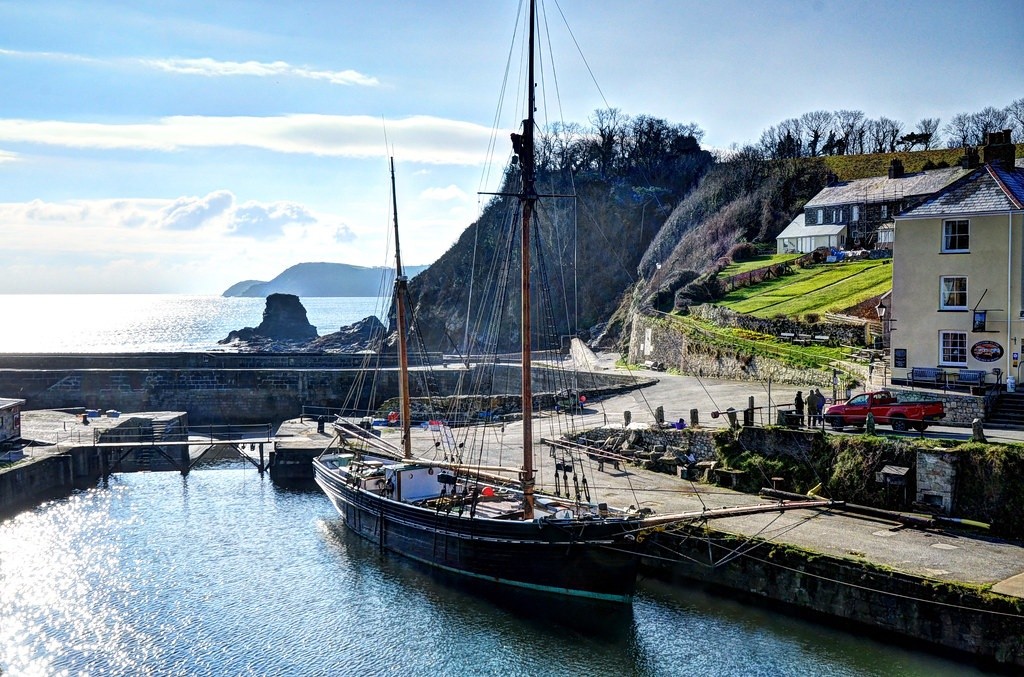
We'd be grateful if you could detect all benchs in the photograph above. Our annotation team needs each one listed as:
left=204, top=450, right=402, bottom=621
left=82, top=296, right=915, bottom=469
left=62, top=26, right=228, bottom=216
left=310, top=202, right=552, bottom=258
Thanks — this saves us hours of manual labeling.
left=846, top=352, right=885, bottom=361
left=810, top=335, right=830, bottom=346
left=947, top=369, right=987, bottom=395
left=793, top=334, right=811, bottom=345
left=640, top=360, right=654, bottom=372
left=651, top=362, right=665, bottom=372
left=906, top=366, right=943, bottom=389
left=778, top=332, right=794, bottom=343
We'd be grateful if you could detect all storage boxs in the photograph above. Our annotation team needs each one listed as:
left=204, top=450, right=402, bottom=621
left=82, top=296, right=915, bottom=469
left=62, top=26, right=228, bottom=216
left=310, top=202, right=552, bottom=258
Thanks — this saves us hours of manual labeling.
left=373, top=418, right=388, bottom=426
left=428, top=419, right=441, bottom=431
left=106, top=411, right=121, bottom=417
left=86, top=409, right=101, bottom=417
left=388, top=412, right=399, bottom=420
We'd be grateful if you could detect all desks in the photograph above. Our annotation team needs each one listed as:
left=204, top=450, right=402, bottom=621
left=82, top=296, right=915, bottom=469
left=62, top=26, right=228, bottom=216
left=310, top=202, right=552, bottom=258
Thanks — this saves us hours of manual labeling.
left=853, top=349, right=883, bottom=363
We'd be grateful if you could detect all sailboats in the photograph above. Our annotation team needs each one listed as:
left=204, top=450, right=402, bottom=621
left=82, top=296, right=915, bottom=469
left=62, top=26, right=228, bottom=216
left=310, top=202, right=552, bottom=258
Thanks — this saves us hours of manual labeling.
left=313, top=2, right=654, bottom=634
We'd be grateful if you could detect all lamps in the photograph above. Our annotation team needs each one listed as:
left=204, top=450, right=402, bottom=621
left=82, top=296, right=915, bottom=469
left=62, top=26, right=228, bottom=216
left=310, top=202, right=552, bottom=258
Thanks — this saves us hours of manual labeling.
left=875, top=299, right=898, bottom=332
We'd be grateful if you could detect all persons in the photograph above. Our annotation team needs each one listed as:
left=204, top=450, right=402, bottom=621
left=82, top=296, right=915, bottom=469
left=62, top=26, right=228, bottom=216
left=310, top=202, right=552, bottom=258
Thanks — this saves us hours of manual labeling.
left=804, top=389, right=821, bottom=429
left=794, top=391, right=806, bottom=426
left=815, top=388, right=825, bottom=425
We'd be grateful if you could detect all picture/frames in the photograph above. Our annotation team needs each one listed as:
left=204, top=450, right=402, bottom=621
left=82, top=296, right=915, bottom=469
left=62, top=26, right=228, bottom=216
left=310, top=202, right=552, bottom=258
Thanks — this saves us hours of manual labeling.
left=13, top=413, right=19, bottom=430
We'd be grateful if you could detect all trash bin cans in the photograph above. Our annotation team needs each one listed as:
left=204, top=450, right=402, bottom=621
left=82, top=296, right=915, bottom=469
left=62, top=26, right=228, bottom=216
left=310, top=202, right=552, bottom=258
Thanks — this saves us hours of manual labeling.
left=1007, top=376, right=1015, bottom=393
left=771, top=478, right=784, bottom=490
left=785, top=409, right=800, bottom=429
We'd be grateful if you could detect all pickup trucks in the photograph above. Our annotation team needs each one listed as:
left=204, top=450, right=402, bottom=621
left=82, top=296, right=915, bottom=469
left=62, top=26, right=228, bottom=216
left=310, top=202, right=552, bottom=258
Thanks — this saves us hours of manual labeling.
left=823, top=390, right=947, bottom=436
